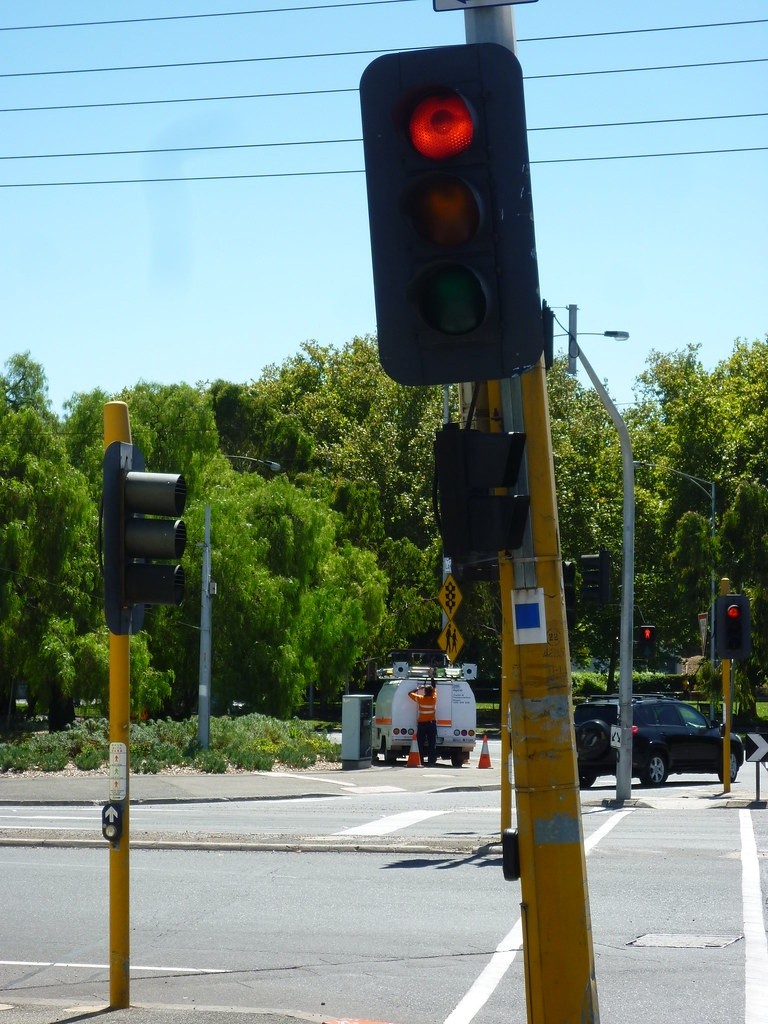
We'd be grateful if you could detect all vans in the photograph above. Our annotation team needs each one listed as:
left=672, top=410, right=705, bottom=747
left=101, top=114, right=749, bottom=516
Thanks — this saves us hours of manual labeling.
left=371, top=662, right=477, bottom=766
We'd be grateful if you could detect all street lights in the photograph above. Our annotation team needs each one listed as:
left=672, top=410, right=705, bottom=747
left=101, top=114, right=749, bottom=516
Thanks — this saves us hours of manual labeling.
left=634, top=455, right=718, bottom=729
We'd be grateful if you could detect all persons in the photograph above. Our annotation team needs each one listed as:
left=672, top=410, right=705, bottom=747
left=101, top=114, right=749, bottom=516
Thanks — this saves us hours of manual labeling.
left=408, top=672, right=438, bottom=767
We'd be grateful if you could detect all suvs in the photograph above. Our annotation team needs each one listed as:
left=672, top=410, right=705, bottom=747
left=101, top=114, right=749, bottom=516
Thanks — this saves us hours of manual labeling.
left=570, top=690, right=745, bottom=793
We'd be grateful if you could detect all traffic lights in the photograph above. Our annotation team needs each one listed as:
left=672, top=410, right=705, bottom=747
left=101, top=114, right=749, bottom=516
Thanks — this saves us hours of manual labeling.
left=108, top=440, right=192, bottom=635
left=357, top=33, right=556, bottom=393
left=637, top=623, right=655, bottom=657
left=721, top=591, right=745, bottom=651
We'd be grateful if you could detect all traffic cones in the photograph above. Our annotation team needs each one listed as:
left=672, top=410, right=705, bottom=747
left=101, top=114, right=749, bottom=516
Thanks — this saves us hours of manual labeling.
left=476, top=734, right=494, bottom=769
left=406, top=731, right=421, bottom=767
